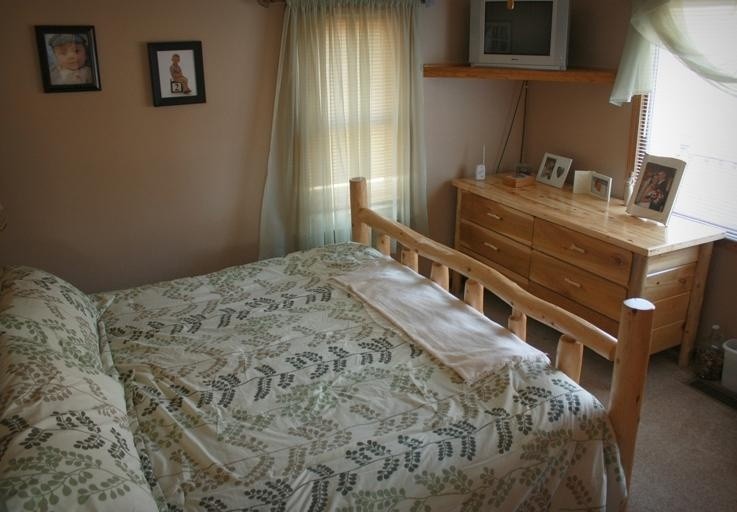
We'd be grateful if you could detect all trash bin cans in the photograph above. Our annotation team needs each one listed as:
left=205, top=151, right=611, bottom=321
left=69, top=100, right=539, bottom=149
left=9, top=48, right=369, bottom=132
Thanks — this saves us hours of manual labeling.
left=720, top=338, right=737, bottom=393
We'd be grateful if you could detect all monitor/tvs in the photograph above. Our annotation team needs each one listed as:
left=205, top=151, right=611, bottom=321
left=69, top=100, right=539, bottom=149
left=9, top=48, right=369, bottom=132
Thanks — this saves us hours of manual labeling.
left=468, top=0, right=570, bottom=71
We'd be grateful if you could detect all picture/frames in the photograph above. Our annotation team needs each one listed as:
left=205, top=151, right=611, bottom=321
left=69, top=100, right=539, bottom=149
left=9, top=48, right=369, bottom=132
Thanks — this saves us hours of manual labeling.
left=147, top=40, right=207, bottom=107
left=33, top=24, right=103, bottom=93
left=535, top=153, right=687, bottom=227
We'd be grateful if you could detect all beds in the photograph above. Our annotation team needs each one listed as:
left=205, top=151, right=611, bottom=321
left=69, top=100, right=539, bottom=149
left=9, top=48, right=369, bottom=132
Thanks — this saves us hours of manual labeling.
left=0, top=177, right=655, bottom=512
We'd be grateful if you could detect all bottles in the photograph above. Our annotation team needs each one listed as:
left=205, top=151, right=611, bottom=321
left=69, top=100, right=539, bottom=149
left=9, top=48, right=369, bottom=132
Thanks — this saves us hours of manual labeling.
left=700, top=324, right=723, bottom=379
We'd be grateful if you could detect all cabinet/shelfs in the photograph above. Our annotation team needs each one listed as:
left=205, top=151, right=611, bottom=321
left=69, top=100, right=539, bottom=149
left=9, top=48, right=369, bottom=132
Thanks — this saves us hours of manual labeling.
left=450, top=168, right=727, bottom=367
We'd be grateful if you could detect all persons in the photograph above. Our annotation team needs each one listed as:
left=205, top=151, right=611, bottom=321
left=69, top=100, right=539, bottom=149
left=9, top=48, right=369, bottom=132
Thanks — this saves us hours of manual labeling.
left=46, top=32, right=92, bottom=85
left=649, top=168, right=668, bottom=212
left=592, top=177, right=602, bottom=195
left=169, top=53, right=193, bottom=93
left=540, top=158, right=555, bottom=180
left=635, top=173, right=660, bottom=209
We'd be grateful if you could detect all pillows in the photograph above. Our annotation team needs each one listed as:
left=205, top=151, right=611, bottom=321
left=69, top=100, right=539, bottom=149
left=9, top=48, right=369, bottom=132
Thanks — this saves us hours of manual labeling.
left=2, top=268, right=136, bottom=385
left=0, top=335, right=169, bottom=511
